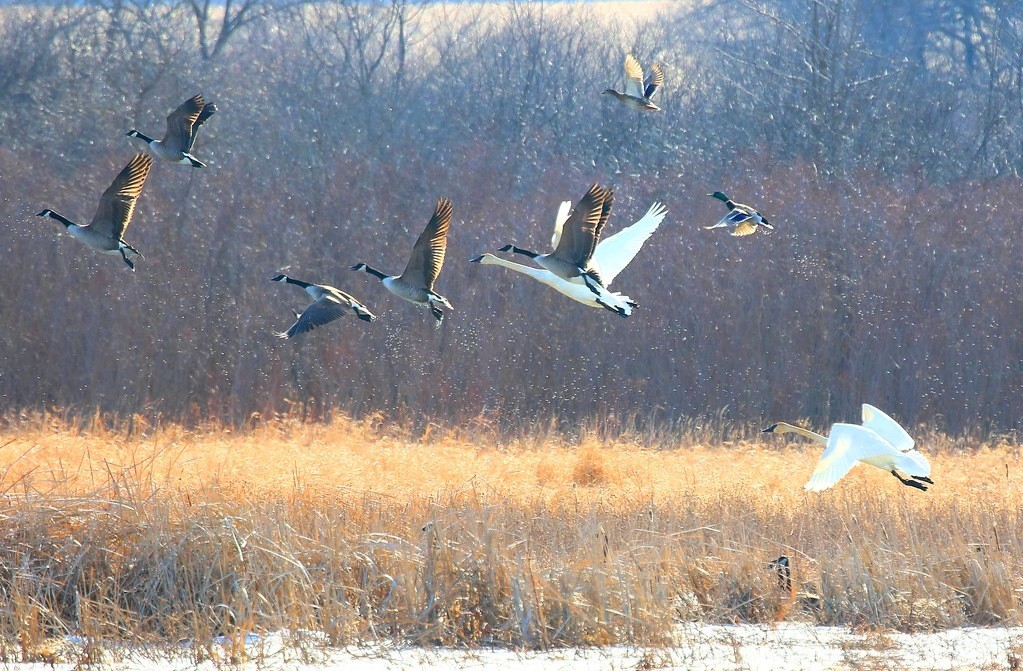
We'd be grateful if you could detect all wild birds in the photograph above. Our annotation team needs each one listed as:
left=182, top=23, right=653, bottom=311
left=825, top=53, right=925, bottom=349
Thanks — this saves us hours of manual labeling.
left=468, top=182, right=669, bottom=318
left=770, top=556, right=821, bottom=613
left=270, top=274, right=377, bottom=339
left=34, top=153, right=153, bottom=271
left=349, top=195, right=454, bottom=321
left=600, top=53, right=663, bottom=111
left=762, top=403, right=934, bottom=492
left=702, top=191, right=774, bottom=237
left=125, top=92, right=219, bottom=169
